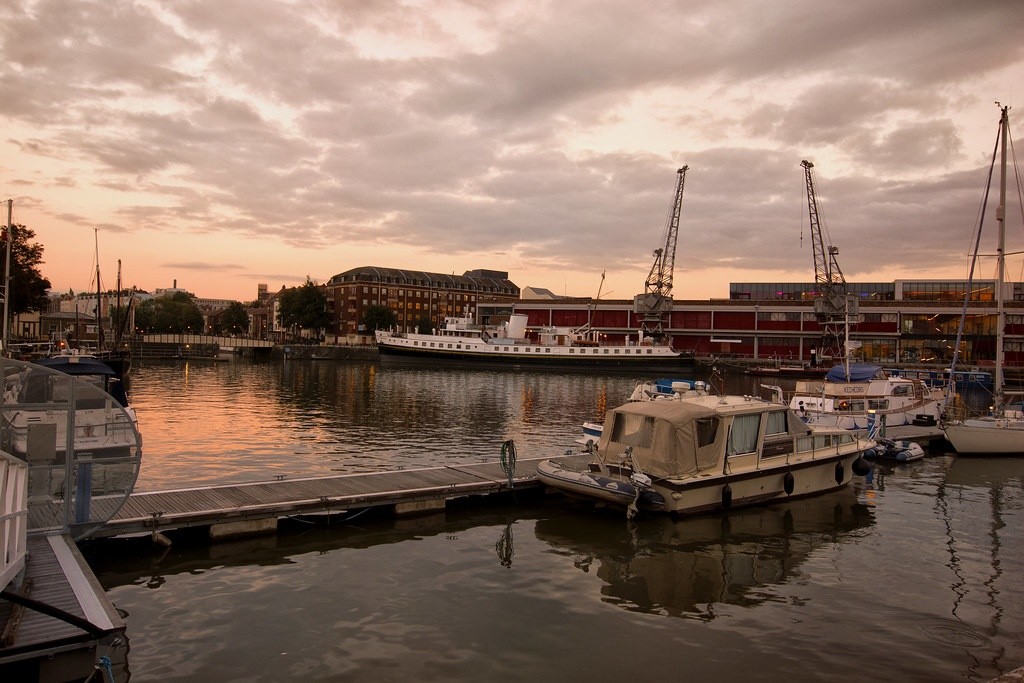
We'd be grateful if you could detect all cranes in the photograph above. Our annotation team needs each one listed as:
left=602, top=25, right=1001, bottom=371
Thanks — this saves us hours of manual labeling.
left=636, top=164, right=688, bottom=346
left=800, top=159, right=863, bottom=365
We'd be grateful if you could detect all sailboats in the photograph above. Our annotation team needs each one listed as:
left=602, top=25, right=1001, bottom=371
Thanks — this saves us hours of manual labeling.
left=52, top=228, right=134, bottom=379
left=936, top=103, right=1024, bottom=456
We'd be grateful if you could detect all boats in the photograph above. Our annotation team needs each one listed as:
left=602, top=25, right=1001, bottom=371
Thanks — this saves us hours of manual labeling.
left=760, top=362, right=956, bottom=430
left=535, top=392, right=876, bottom=526
left=864, top=439, right=924, bottom=463
left=574, top=379, right=711, bottom=447
left=2, top=355, right=142, bottom=461
left=376, top=318, right=713, bottom=372
